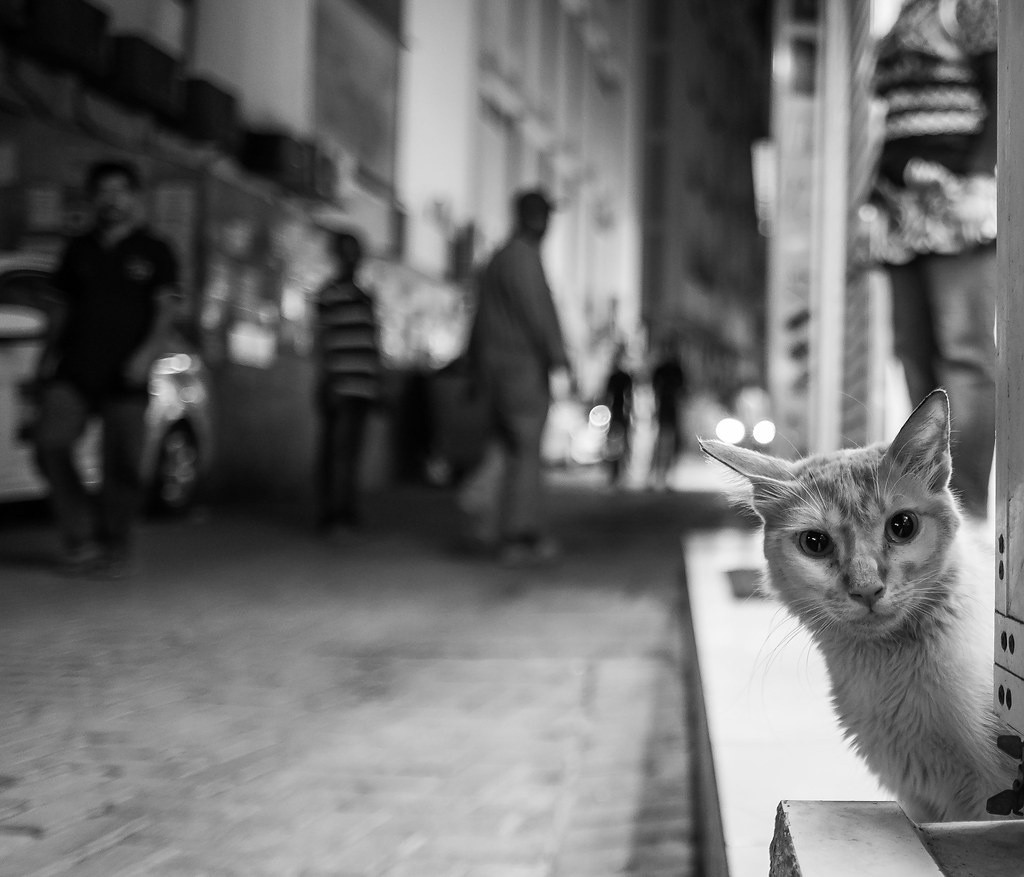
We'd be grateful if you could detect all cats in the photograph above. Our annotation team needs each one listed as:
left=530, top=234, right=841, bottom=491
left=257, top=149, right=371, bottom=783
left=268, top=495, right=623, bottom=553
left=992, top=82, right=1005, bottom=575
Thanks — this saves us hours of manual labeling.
left=696, top=388, right=1024, bottom=822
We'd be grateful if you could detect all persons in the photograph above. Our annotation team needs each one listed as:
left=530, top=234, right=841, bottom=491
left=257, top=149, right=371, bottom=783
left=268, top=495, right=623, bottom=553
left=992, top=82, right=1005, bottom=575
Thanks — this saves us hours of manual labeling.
left=316, top=189, right=680, bottom=566
left=33, top=159, right=186, bottom=580
left=849, top=1, right=1001, bottom=517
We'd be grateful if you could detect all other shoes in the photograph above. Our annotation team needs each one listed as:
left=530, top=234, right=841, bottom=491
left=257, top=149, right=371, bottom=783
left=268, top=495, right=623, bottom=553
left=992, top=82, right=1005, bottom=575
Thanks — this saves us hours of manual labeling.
left=466, top=545, right=520, bottom=568
left=88, top=546, right=137, bottom=578
left=53, top=545, right=92, bottom=575
left=521, top=537, right=560, bottom=561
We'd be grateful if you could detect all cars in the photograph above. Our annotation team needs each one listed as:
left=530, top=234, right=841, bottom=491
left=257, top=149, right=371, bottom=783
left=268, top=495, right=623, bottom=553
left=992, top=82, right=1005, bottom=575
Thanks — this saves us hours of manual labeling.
left=0, top=246, right=225, bottom=527
